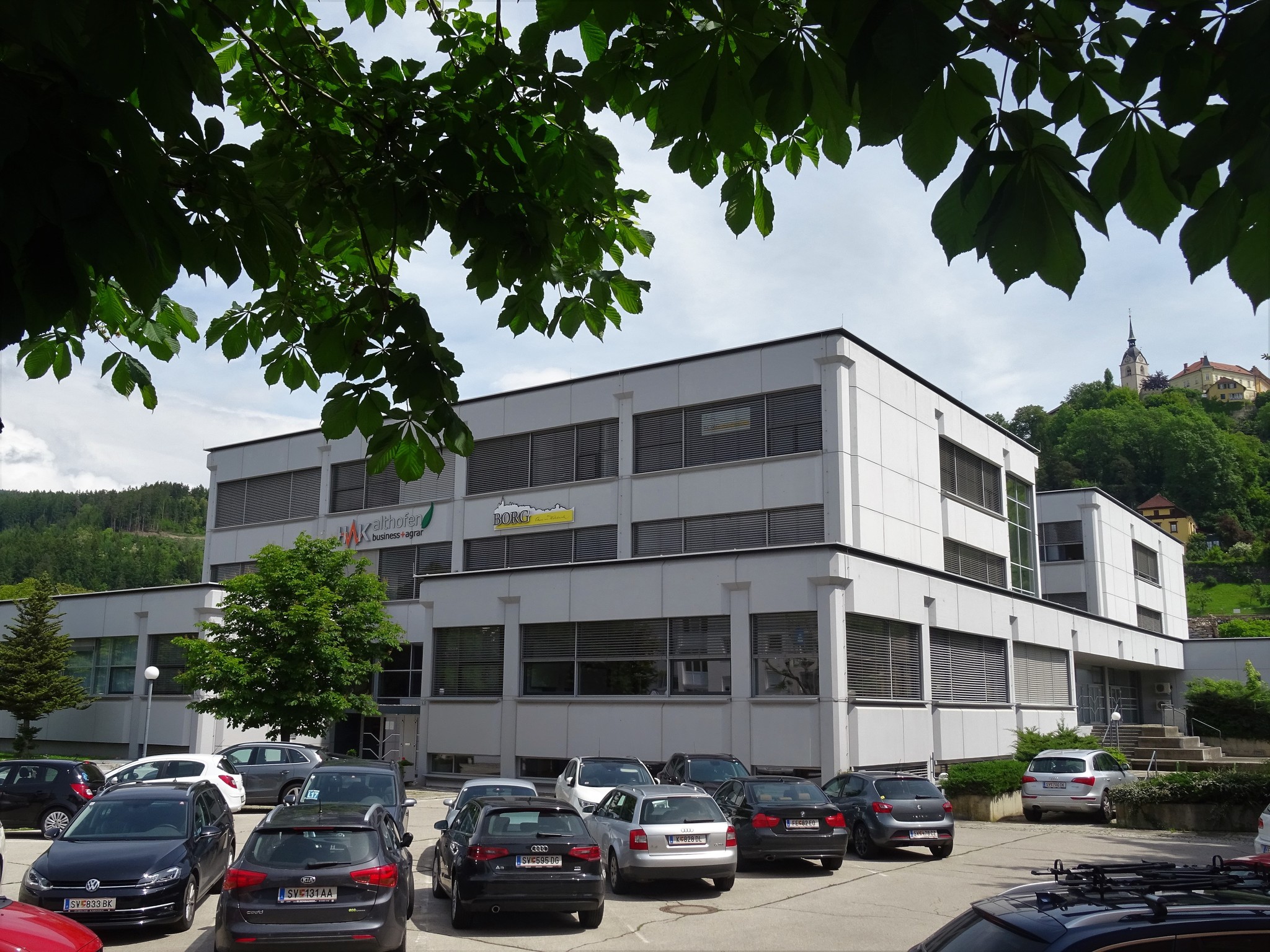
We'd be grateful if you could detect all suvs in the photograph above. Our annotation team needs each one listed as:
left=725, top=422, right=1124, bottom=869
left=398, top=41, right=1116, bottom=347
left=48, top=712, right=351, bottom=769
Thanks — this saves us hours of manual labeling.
left=581, top=782, right=738, bottom=894
left=283, top=758, right=418, bottom=844
left=553, top=755, right=668, bottom=823
left=219, top=741, right=330, bottom=815
left=906, top=852, right=1270, bottom=952
left=213, top=803, right=415, bottom=952
left=657, top=752, right=752, bottom=798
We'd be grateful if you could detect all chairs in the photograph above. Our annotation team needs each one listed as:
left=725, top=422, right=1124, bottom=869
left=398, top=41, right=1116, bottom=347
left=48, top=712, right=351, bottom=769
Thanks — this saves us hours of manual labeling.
left=537, top=812, right=551, bottom=829
left=345, top=831, right=368, bottom=856
left=491, top=817, right=511, bottom=832
left=320, top=776, right=341, bottom=802
left=15, top=767, right=30, bottom=784
left=364, top=777, right=384, bottom=798
left=99, top=812, right=128, bottom=834
left=783, top=790, right=797, bottom=800
left=661, top=800, right=678, bottom=820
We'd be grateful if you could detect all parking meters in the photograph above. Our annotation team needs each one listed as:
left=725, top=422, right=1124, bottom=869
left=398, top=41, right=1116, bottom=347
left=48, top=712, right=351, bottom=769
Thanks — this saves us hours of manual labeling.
left=1111, top=712, right=1121, bottom=753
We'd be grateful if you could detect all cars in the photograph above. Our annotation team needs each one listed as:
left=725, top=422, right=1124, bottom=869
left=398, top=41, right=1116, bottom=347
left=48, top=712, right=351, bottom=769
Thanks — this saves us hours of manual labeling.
left=324, top=752, right=361, bottom=761
left=711, top=775, right=848, bottom=871
left=105, top=753, right=247, bottom=813
left=1254, top=802, right=1270, bottom=855
left=1020, top=749, right=1140, bottom=823
left=431, top=794, right=606, bottom=930
left=820, top=770, right=955, bottom=858
left=0, top=758, right=107, bottom=838
left=0, top=894, right=104, bottom=952
left=17, top=780, right=236, bottom=934
left=443, top=791, right=538, bottom=831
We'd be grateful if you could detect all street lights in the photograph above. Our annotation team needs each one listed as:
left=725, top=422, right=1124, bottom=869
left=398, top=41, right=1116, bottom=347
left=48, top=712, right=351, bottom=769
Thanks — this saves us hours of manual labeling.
left=141, top=666, right=160, bottom=759
